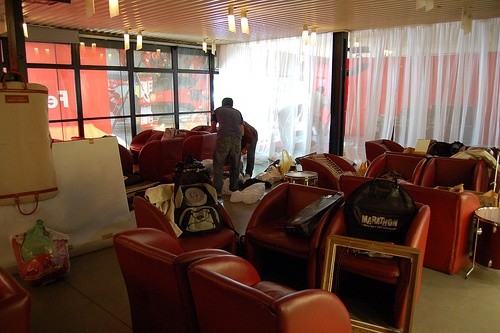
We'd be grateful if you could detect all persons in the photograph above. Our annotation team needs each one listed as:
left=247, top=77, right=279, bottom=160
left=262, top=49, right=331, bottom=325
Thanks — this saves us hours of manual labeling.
left=241, top=121, right=258, bottom=180
left=208, top=98, right=244, bottom=197
left=311, top=86, right=326, bottom=148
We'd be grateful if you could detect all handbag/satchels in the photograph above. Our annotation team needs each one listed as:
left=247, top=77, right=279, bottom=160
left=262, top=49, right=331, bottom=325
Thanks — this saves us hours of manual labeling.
left=344, top=169, right=416, bottom=241
left=228, top=150, right=295, bottom=204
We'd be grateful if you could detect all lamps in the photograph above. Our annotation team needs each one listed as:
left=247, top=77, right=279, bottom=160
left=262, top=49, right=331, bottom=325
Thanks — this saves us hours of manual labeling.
left=136, top=31, right=143, bottom=50
left=23, top=17, right=28, bottom=38
left=85, top=0, right=95, bottom=19
left=109, top=0, right=119, bottom=18
left=240, top=9, right=249, bottom=34
left=211, top=40, right=216, bottom=56
left=202, top=39, right=207, bottom=53
left=227, top=5, right=236, bottom=33
left=124, top=30, right=130, bottom=51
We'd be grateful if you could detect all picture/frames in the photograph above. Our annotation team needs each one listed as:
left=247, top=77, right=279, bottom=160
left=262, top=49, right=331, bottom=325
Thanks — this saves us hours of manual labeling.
left=320, top=236, right=421, bottom=333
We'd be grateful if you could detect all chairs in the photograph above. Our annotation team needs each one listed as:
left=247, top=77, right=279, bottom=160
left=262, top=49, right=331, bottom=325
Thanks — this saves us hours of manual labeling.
left=51, top=126, right=500, bottom=333
left=0, top=265, right=32, bottom=333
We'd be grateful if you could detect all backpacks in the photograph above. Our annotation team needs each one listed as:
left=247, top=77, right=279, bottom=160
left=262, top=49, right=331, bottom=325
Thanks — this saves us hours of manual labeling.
left=174, top=173, right=222, bottom=233
